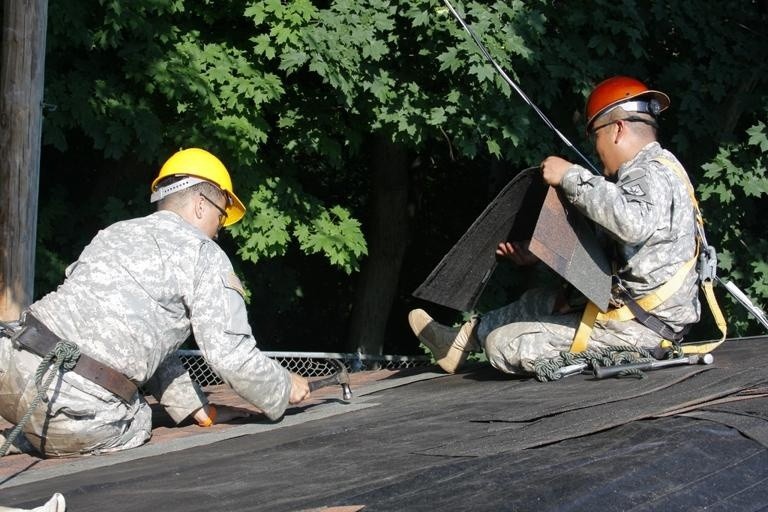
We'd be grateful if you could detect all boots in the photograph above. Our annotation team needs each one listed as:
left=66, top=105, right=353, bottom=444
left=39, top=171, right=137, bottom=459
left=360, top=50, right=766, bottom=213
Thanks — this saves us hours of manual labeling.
left=406, top=303, right=481, bottom=377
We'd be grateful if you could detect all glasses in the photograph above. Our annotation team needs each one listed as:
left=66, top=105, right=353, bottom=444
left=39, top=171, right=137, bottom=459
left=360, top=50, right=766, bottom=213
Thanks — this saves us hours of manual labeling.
left=199, top=191, right=229, bottom=226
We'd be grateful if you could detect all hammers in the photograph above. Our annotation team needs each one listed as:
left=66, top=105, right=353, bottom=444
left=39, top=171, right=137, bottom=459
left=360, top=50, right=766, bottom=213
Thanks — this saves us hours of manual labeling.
left=308, top=358, right=353, bottom=400
left=592, top=353, right=714, bottom=379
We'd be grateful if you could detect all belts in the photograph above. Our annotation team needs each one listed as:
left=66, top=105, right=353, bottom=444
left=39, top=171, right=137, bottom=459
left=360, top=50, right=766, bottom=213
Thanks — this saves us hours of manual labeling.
left=10, top=308, right=139, bottom=407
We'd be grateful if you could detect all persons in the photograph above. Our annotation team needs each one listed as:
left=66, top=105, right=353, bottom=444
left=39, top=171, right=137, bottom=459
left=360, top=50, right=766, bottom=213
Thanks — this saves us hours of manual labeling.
left=0, top=147, right=311, bottom=459
left=407, top=77, right=702, bottom=378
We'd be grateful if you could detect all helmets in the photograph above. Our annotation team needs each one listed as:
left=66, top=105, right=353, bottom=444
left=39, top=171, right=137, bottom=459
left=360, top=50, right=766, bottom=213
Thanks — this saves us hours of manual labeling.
left=580, top=74, right=672, bottom=138
left=148, top=147, right=247, bottom=228
